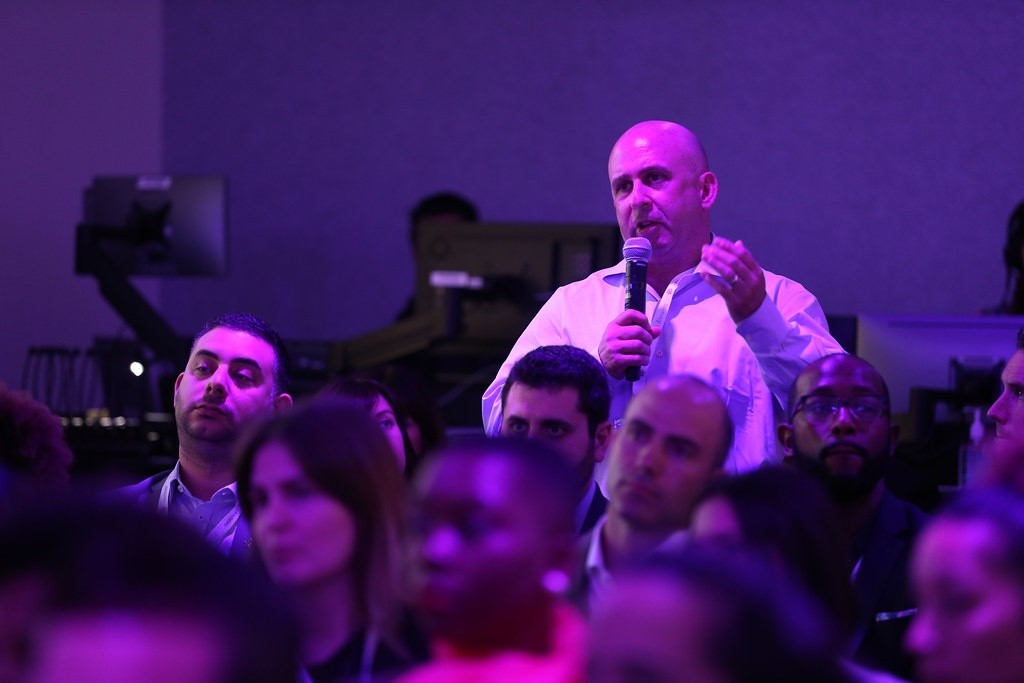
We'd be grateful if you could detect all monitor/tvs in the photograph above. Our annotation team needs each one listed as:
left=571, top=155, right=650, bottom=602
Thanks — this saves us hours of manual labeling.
left=856, top=313, right=1024, bottom=426
left=84, top=174, right=232, bottom=277
left=415, top=221, right=624, bottom=354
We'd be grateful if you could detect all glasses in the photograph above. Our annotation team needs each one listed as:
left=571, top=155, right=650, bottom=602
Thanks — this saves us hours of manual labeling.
left=789, top=394, right=891, bottom=423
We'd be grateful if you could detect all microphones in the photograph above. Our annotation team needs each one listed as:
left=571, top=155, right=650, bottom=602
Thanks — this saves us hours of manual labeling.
left=621, top=237, right=653, bottom=381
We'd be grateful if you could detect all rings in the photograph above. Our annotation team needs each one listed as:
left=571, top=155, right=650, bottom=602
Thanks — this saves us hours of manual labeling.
left=732, top=275, right=738, bottom=283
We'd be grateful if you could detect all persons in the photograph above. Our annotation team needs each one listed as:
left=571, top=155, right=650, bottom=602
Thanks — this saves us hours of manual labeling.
left=479, top=120, right=851, bottom=474
left=396, top=191, right=480, bottom=326
left=0, top=312, right=1024, bottom=683
